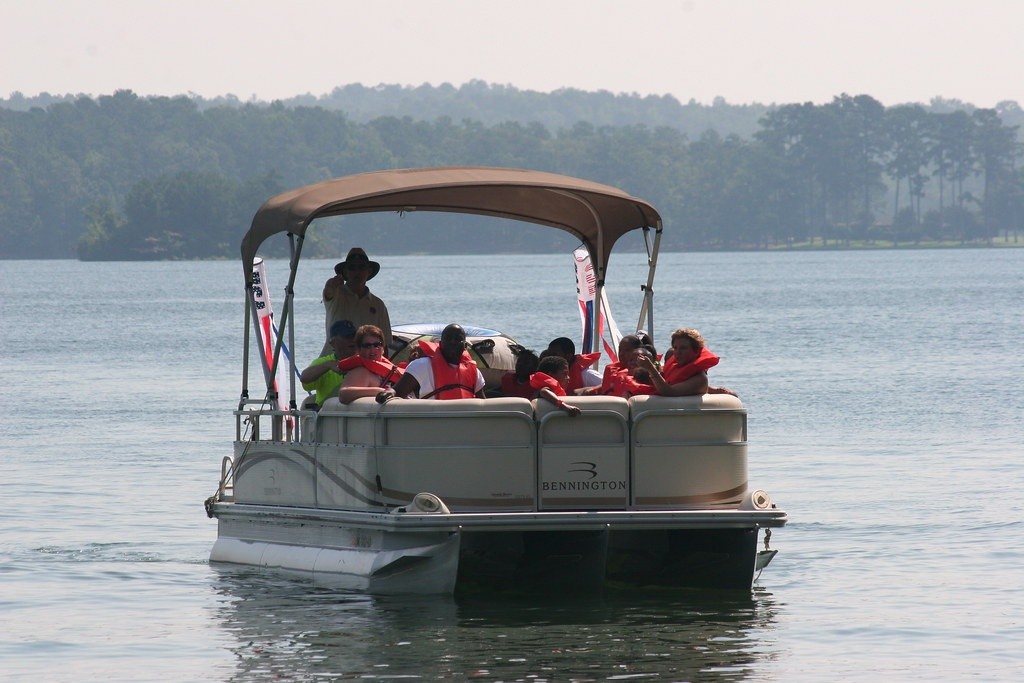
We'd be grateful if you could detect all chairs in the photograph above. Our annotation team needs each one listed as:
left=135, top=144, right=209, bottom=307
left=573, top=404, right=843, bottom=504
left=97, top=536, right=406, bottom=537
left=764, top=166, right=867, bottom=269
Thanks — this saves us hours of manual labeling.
left=628, top=395, right=747, bottom=506
left=531, top=395, right=630, bottom=510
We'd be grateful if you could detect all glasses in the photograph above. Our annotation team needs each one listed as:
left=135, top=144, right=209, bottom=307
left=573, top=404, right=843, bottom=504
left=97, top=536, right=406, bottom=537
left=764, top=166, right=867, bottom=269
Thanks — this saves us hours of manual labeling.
left=348, top=265, right=368, bottom=270
left=362, top=341, right=382, bottom=349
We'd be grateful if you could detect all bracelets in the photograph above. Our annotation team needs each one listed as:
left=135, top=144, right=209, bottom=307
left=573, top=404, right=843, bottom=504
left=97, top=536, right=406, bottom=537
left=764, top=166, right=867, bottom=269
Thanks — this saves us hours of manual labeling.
left=555, top=400, right=564, bottom=408
left=648, top=372, right=661, bottom=379
left=593, top=386, right=599, bottom=395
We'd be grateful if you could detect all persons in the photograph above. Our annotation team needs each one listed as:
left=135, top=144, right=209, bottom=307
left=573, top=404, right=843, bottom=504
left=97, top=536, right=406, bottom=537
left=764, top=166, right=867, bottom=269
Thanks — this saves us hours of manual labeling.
left=318, top=248, right=393, bottom=359
left=301, top=320, right=708, bottom=418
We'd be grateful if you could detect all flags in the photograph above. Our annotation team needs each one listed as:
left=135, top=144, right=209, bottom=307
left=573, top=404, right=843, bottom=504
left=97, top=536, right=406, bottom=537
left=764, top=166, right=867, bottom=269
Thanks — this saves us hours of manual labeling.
left=571, top=243, right=624, bottom=362
left=245, top=256, right=295, bottom=428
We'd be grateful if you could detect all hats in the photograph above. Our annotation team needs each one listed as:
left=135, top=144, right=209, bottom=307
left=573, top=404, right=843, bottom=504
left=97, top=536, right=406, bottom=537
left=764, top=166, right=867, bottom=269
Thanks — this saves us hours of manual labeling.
left=335, top=248, right=380, bottom=281
left=330, top=320, right=356, bottom=337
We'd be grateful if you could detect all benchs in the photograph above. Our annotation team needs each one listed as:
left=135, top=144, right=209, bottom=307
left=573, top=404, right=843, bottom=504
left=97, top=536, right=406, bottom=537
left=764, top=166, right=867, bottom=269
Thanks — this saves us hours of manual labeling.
left=306, top=389, right=538, bottom=512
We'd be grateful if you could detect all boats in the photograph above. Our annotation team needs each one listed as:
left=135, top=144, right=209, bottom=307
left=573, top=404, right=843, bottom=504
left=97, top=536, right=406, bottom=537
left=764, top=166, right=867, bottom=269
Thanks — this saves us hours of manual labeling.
left=204, top=164, right=787, bottom=601
